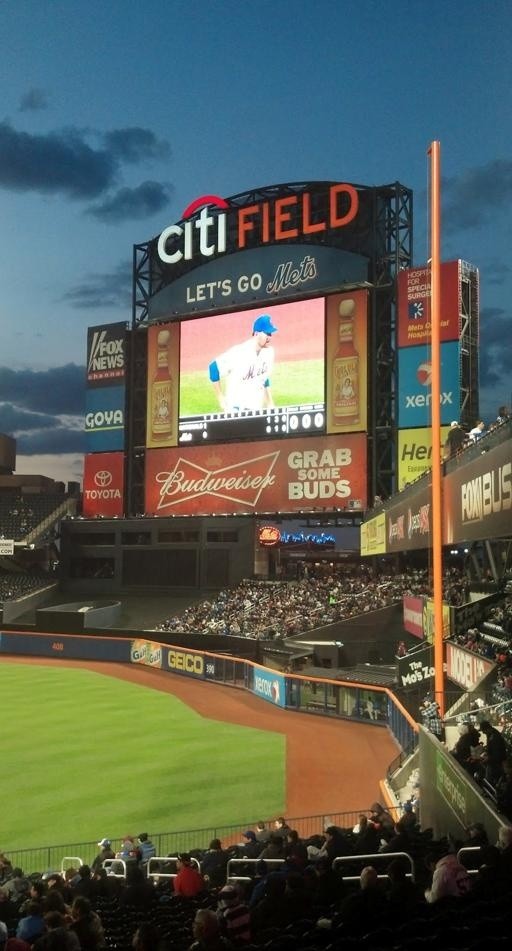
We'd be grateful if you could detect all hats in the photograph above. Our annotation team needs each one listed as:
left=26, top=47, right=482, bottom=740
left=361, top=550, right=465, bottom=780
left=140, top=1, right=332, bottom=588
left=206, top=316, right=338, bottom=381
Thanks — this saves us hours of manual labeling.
left=98, top=839, right=111, bottom=847
left=422, top=696, right=433, bottom=702
left=244, top=830, right=257, bottom=841
left=252, top=314, right=278, bottom=334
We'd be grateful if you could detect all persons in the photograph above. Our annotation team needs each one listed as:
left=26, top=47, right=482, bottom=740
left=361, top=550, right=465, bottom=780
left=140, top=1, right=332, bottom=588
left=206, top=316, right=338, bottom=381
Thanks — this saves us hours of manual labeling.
left=158, top=400, right=169, bottom=417
left=156, top=559, right=512, bottom=802
left=444, top=407, right=510, bottom=456
left=7, top=494, right=34, bottom=531
left=0, top=801, right=511, bottom=951
left=208, top=315, right=278, bottom=412
left=341, top=378, right=354, bottom=399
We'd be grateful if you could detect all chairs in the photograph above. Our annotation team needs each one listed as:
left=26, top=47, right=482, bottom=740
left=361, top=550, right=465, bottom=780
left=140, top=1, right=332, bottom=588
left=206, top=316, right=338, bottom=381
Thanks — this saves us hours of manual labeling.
left=1, top=825, right=512, bottom=951
